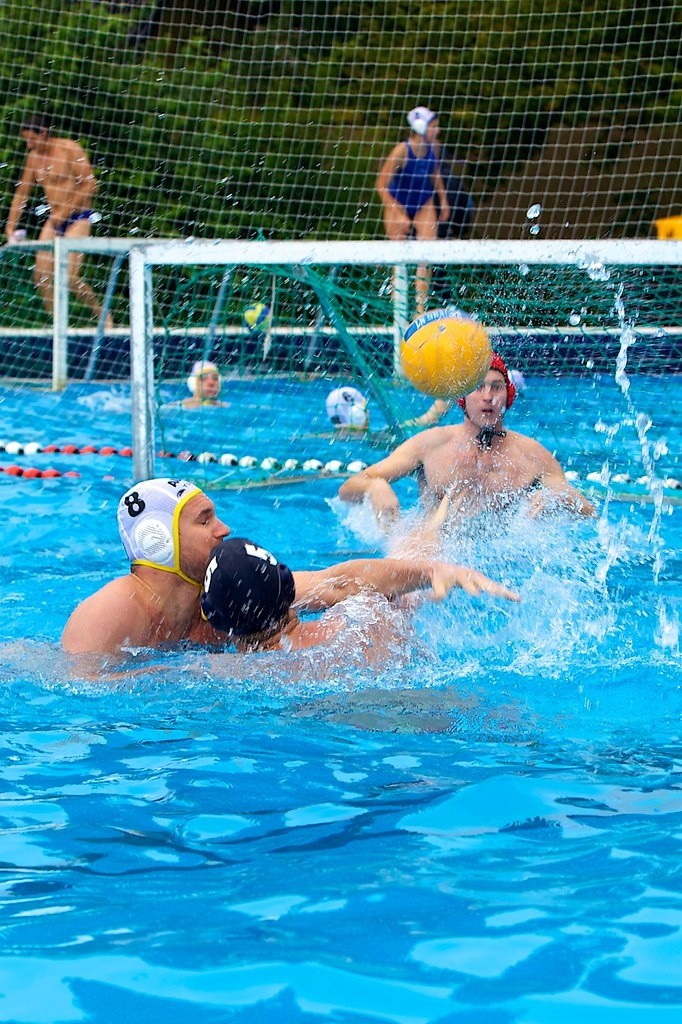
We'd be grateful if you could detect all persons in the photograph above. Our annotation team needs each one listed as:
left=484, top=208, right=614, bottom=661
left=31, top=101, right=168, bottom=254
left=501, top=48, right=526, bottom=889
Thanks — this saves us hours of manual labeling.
left=199, top=538, right=346, bottom=653
left=375, top=106, right=450, bottom=320
left=160, top=361, right=231, bottom=411
left=62, top=478, right=518, bottom=652
left=6, top=116, right=113, bottom=330
left=338, top=353, right=595, bottom=516
left=326, top=387, right=453, bottom=429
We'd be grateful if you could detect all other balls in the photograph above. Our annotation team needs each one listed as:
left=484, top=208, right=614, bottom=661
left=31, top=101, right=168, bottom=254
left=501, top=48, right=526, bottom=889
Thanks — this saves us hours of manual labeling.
left=399, top=307, right=495, bottom=402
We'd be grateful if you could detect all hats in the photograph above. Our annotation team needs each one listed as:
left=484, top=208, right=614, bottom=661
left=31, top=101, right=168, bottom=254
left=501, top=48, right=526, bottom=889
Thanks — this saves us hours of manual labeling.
left=202, top=538, right=296, bottom=636
left=457, top=352, right=515, bottom=413
left=407, top=106, right=437, bottom=136
left=326, top=389, right=369, bottom=431
left=115, top=478, right=203, bottom=575
left=187, top=361, right=223, bottom=399
